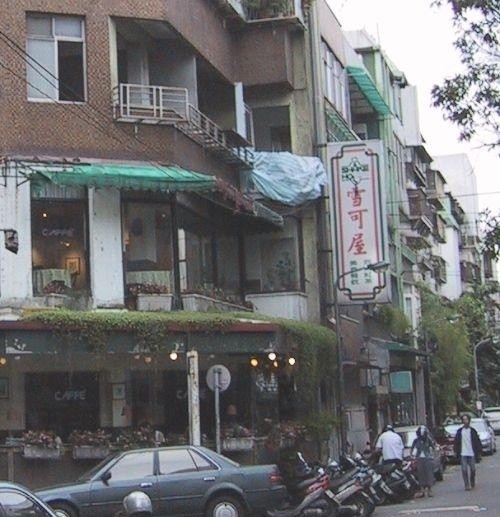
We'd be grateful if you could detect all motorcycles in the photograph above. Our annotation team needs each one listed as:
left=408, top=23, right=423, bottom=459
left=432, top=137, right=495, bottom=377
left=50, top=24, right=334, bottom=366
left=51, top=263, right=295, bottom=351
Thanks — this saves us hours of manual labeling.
left=293, top=450, right=418, bottom=516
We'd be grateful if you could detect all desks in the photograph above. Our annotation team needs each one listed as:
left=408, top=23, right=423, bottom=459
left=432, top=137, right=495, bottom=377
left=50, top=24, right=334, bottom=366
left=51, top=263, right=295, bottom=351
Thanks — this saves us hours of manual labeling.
left=33, top=268, right=70, bottom=294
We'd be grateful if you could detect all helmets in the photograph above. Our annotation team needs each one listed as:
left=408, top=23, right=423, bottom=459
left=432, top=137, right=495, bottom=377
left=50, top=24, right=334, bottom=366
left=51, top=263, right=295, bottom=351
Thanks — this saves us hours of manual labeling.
left=416, top=426, right=427, bottom=438
left=383, top=425, right=394, bottom=432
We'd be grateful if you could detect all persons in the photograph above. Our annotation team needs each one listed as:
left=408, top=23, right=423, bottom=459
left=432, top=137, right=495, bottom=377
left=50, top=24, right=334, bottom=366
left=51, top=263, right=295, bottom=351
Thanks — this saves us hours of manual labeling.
left=453, top=414, right=483, bottom=491
left=135, top=422, right=166, bottom=447
left=374, top=424, right=404, bottom=469
left=410, top=426, right=435, bottom=498
left=225, top=405, right=243, bottom=430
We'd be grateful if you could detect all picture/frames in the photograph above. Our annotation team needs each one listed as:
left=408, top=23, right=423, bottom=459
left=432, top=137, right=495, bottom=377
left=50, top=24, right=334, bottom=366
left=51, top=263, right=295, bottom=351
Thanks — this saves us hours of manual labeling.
left=65, top=257, right=80, bottom=275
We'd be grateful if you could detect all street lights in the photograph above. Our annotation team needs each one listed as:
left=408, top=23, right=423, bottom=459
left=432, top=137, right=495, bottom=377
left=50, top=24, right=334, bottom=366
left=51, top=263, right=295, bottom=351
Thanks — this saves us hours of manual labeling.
left=333, top=261, right=390, bottom=465
left=473, top=336, right=500, bottom=417
left=417, top=314, right=462, bottom=429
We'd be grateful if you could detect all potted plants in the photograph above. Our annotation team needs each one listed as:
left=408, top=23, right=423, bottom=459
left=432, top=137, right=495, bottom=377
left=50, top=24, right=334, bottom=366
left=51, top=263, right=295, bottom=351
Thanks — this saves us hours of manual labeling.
left=127, top=281, right=173, bottom=311
left=222, top=423, right=255, bottom=451
left=182, top=279, right=253, bottom=314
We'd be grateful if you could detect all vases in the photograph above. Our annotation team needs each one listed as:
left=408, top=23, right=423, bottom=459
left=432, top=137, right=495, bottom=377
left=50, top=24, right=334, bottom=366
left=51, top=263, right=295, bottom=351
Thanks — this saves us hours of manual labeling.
left=25, top=446, right=112, bottom=461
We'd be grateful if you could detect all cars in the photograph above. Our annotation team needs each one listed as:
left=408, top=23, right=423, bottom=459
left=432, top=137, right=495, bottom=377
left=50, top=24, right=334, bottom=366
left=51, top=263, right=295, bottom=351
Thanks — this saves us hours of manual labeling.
left=442, top=417, right=496, bottom=466
left=0, top=445, right=287, bottom=516
left=482, top=406, right=500, bottom=433
left=379, top=423, right=446, bottom=484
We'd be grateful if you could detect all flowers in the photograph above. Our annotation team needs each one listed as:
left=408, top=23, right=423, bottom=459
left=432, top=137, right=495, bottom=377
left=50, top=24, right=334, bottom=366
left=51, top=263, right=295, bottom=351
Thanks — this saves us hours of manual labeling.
left=24, top=422, right=158, bottom=447
